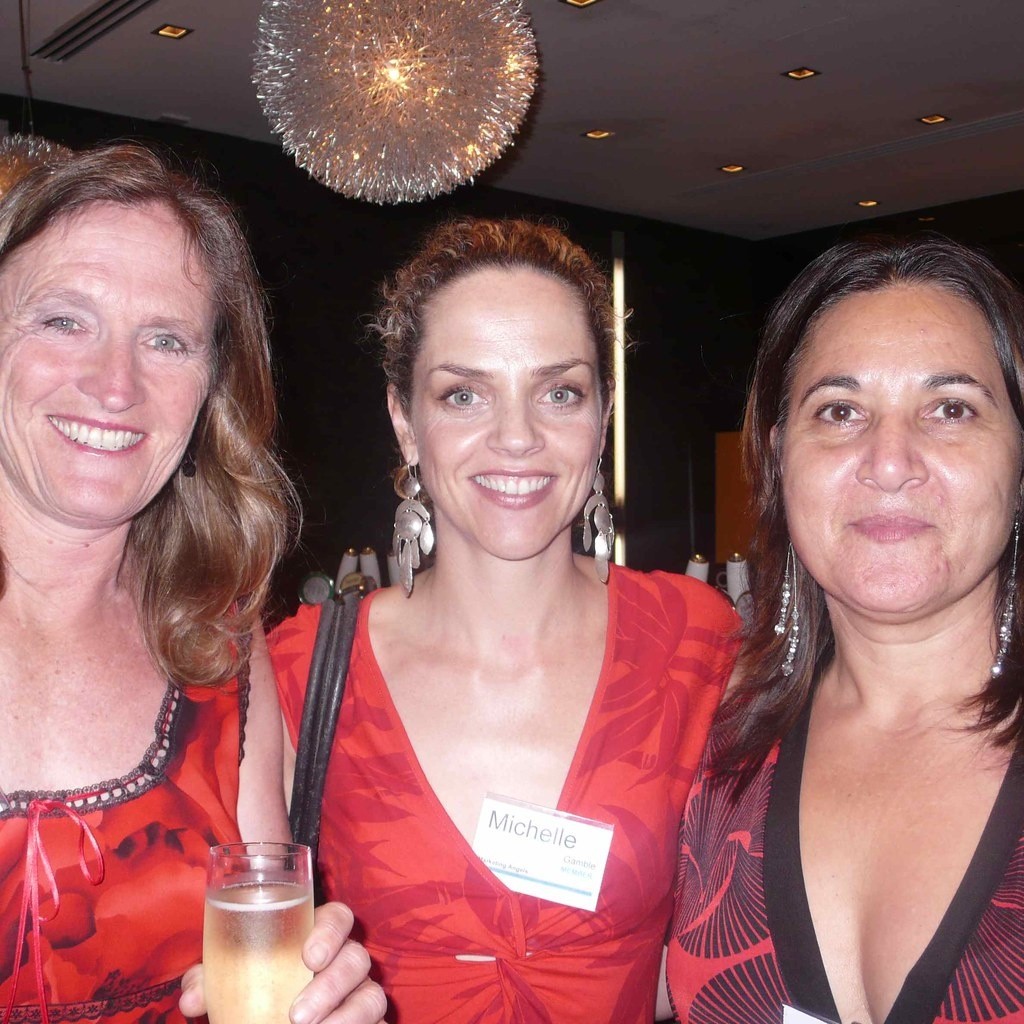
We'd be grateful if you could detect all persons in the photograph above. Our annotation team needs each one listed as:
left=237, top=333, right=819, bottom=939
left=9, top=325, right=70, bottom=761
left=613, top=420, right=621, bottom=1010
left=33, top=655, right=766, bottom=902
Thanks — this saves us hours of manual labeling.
left=1, top=143, right=391, bottom=1024
left=260, top=217, right=758, bottom=1024
left=665, top=231, right=1023, bottom=1024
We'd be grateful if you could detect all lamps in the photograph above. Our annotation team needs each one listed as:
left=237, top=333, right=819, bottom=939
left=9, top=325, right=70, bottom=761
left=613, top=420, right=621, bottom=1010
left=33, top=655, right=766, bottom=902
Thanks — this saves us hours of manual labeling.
left=246, top=0, right=541, bottom=206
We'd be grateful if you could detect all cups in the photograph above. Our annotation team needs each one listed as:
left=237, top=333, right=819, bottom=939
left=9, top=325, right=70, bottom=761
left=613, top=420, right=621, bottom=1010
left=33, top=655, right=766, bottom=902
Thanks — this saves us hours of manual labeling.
left=204, top=841, right=314, bottom=1024
